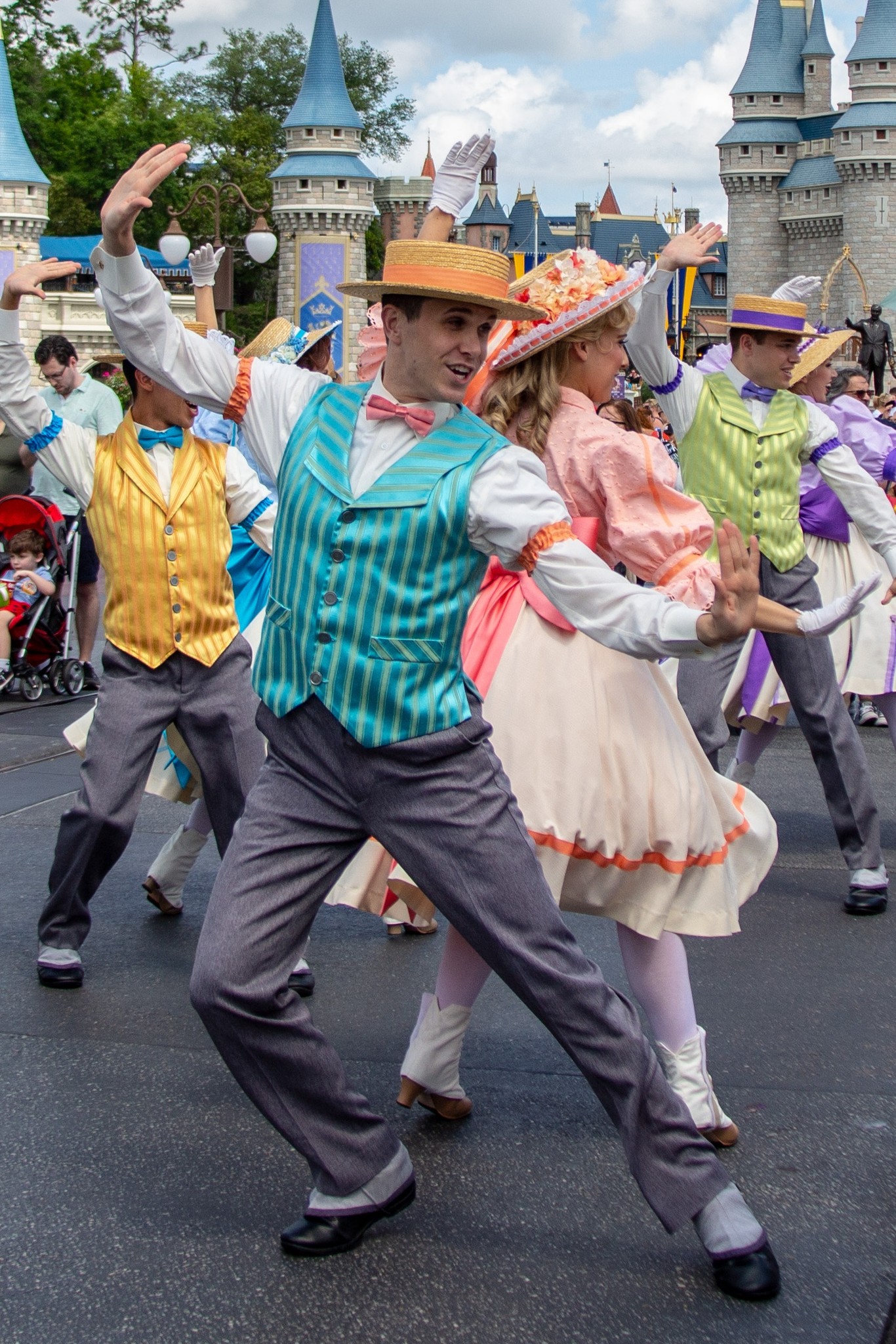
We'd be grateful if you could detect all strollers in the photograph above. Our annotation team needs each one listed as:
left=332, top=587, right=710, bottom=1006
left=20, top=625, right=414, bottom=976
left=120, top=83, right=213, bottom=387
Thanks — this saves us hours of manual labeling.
left=0, top=484, right=88, bottom=704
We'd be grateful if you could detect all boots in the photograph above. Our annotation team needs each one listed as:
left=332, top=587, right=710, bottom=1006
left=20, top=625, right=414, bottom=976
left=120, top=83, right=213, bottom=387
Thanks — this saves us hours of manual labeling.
left=142, top=825, right=208, bottom=916
left=655, top=1024, right=740, bottom=1146
left=396, top=992, right=472, bottom=1120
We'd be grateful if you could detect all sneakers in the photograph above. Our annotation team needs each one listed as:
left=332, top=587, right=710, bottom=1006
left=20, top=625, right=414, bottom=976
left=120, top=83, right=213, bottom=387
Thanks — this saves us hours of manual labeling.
left=858, top=701, right=889, bottom=728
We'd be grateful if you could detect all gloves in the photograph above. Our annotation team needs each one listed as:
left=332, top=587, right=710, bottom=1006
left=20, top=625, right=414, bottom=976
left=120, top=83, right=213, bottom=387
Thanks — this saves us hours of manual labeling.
left=771, top=276, right=821, bottom=301
left=795, top=572, right=884, bottom=638
left=188, top=243, right=225, bottom=288
left=427, top=133, right=496, bottom=219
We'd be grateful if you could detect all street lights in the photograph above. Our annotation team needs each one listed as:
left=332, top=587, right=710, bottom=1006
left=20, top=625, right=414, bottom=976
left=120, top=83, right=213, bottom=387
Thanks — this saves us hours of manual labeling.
left=158, top=182, right=279, bottom=333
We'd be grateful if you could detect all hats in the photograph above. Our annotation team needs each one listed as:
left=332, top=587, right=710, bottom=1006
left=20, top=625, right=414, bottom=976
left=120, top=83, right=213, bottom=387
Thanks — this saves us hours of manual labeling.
left=488, top=248, right=646, bottom=372
left=705, top=294, right=830, bottom=340
left=237, top=317, right=342, bottom=365
left=336, top=239, right=548, bottom=321
left=93, top=321, right=208, bottom=364
left=789, top=321, right=857, bottom=387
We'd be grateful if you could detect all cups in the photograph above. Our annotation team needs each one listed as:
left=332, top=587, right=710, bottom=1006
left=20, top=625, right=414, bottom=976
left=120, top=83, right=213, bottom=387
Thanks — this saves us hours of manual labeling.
left=0, top=579, right=16, bottom=607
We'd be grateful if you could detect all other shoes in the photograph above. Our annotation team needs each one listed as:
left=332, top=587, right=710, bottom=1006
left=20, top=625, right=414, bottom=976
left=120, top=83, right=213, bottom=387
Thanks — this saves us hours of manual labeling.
left=0, top=666, right=14, bottom=688
left=84, top=661, right=101, bottom=686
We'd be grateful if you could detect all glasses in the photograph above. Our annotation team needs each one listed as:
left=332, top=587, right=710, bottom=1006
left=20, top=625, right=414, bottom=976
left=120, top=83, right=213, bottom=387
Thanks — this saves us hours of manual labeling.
left=38, top=363, right=67, bottom=382
left=842, top=390, right=875, bottom=399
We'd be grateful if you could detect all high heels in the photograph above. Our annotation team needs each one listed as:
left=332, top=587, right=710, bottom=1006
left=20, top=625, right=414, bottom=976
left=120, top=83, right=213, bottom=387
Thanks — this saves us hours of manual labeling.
left=383, top=916, right=438, bottom=936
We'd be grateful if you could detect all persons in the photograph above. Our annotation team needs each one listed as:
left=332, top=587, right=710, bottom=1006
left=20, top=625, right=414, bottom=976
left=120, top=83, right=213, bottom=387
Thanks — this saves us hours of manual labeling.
left=89, top=140, right=779, bottom=1301
left=0, top=219, right=896, bottom=986
left=396, top=132, right=883, bottom=1151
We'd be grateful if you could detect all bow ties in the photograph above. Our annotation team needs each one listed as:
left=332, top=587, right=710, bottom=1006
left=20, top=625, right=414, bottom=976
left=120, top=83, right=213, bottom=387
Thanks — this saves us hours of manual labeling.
left=741, top=380, right=777, bottom=404
left=366, top=394, right=436, bottom=437
left=137, top=424, right=184, bottom=450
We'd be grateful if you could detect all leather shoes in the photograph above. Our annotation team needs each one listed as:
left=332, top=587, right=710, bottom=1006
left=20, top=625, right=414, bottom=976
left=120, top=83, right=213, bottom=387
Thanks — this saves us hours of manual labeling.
left=288, top=974, right=315, bottom=995
left=711, top=1239, right=782, bottom=1303
left=37, top=964, right=85, bottom=989
left=281, top=1178, right=417, bottom=1257
left=841, top=886, right=888, bottom=916
